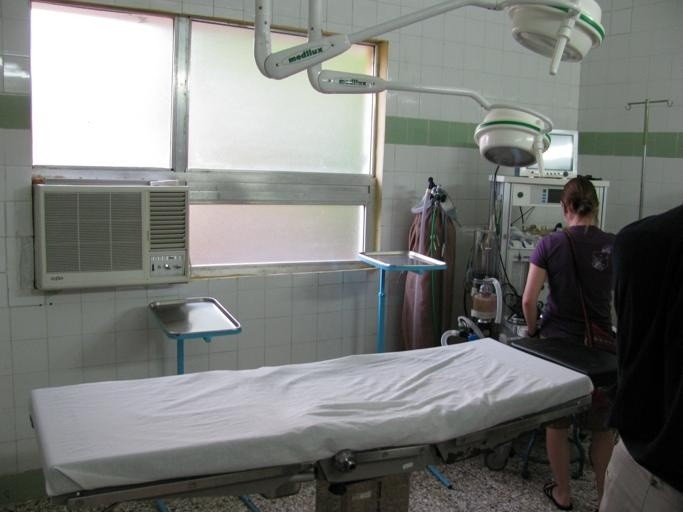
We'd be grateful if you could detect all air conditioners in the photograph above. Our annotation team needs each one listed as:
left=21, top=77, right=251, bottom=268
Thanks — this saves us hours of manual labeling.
left=30, top=182, right=190, bottom=291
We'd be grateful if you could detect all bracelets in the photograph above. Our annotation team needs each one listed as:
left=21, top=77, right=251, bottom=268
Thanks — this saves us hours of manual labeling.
left=528, top=329, right=539, bottom=338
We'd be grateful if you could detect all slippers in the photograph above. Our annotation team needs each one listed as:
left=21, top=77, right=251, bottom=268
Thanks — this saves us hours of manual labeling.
left=543, top=482, right=573, bottom=511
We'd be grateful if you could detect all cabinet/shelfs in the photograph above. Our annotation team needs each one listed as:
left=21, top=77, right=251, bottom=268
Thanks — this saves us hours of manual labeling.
left=488, top=173, right=611, bottom=285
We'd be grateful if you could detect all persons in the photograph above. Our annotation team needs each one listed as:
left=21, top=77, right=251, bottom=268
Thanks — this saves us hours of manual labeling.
left=598, top=202, right=682, bottom=512
left=523, top=177, right=616, bottom=512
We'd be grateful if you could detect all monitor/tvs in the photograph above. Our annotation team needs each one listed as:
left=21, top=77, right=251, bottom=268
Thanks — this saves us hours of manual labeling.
left=518, top=129, right=579, bottom=178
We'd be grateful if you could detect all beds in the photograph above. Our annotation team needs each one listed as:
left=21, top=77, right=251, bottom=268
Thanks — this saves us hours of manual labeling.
left=28, top=323, right=624, bottom=511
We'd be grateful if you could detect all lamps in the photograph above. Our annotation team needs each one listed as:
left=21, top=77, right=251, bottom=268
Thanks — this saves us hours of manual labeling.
left=304, top=0, right=557, bottom=177
left=254, top=1, right=606, bottom=82
